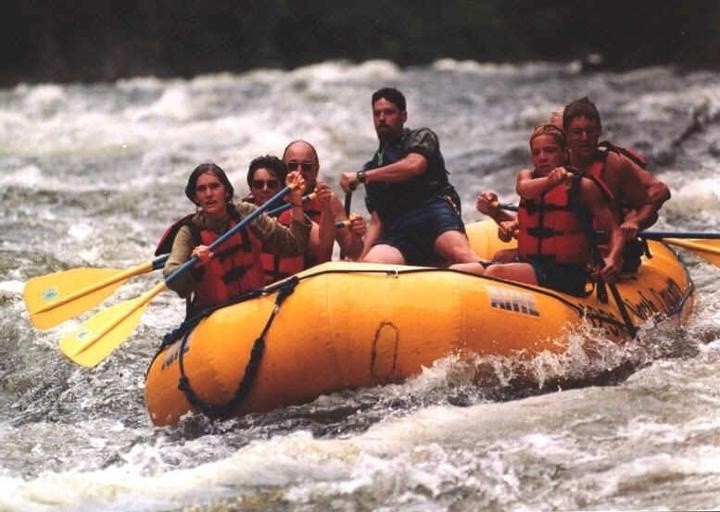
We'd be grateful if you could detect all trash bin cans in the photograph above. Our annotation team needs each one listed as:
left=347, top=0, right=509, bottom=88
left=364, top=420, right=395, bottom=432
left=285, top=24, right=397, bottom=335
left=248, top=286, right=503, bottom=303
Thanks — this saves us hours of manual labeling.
left=356, top=171, right=366, bottom=183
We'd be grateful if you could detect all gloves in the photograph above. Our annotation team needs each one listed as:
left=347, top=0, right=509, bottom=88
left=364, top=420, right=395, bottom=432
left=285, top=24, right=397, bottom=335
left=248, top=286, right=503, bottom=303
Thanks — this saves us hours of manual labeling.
left=142, top=210, right=698, bottom=435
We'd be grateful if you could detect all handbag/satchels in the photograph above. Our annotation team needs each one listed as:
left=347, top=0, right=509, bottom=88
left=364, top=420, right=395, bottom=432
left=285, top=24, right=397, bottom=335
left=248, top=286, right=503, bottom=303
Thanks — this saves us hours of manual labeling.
left=291, top=202, right=304, bottom=208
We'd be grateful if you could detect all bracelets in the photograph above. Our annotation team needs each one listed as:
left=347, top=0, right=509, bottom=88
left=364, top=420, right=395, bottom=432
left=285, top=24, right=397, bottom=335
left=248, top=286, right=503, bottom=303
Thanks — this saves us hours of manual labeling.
left=291, top=202, right=304, bottom=208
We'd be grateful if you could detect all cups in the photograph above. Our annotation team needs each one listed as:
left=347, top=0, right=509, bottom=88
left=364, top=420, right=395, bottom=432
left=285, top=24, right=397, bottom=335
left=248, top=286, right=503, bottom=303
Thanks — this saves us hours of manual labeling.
left=286, top=162, right=315, bottom=172
left=252, top=179, right=279, bottom=190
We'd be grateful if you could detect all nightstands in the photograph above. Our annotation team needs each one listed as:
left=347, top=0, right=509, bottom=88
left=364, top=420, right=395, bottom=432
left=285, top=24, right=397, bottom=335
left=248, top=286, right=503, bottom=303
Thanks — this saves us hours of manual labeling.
left=58, top=184, right=306, bottom=370
left=487, top=196, right=720, bottom=269
left=22, top=188, right=320, bottom=330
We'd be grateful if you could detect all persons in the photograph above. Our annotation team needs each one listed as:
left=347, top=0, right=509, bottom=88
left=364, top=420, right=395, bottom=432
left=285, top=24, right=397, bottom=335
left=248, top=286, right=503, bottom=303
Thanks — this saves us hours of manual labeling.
left=476, top=98, right=669, bottom=274
left=163, top=162, right=314, bottom=320
left=282, top=140, right=367, bottom=261
left=448, top=124, right=623, bottom=295
left=339, top=87, right=490, bottom=266
left=241, top=154, right=337, bottom=266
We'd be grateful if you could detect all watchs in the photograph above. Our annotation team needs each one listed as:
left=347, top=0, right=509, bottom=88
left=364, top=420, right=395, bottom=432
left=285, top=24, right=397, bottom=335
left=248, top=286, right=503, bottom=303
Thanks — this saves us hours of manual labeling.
left=356, top=171, right=366, bottom=183
